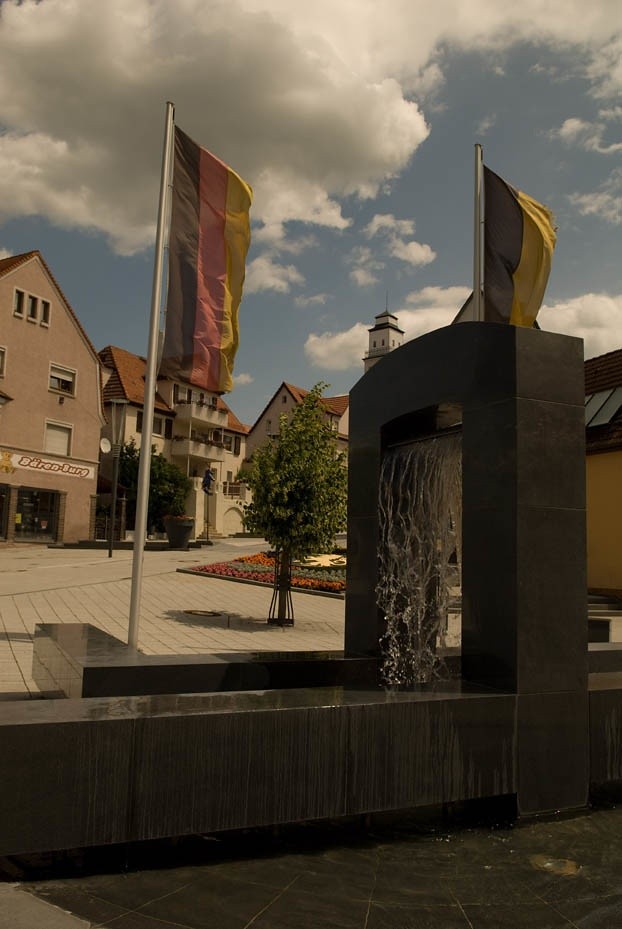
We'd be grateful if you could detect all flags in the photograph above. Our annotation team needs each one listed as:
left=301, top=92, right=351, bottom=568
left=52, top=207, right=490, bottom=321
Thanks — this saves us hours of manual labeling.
left=157, top=118, right=257, bottom=398
left=481, top=162, right=557, bottom=325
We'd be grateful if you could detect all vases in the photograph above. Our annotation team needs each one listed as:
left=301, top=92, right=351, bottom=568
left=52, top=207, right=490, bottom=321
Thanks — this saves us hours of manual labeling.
left=163, top=523, right=193, bottom=551
